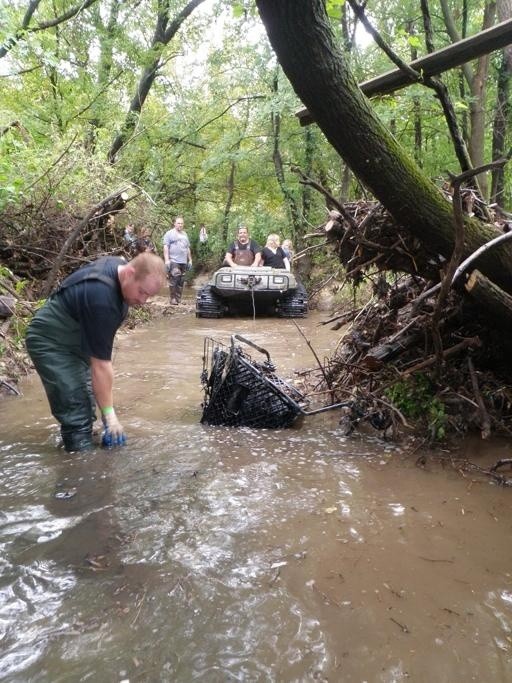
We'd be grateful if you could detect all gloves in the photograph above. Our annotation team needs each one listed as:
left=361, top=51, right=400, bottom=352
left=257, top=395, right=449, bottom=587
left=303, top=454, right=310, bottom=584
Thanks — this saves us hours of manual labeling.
left=164, top=261, right=171, bottom=273
left=187, top=261, right=192, bottom=272
left=102, top=407, right=126, bottom=445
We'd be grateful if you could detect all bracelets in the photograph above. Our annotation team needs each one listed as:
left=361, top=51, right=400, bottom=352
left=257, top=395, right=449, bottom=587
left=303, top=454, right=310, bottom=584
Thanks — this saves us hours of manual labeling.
left=100, top=407, right=115, bottom=415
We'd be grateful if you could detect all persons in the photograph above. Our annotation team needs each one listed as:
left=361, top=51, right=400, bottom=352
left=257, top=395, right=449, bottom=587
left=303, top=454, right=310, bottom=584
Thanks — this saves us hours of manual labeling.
left=281, top=238, right=294, bottom=259
left=259, top=233, right=291, bottom=269
left=163, top=216, right=192, bottom=305
left=225, top=224, right=262, bottom=267
left=199, top=223, right=207, bottom=243
left=122, top=224, right=138, bottom=246
left=139, top=227, right=156, bottom=252
left=24, top=251, right=167, bottom=450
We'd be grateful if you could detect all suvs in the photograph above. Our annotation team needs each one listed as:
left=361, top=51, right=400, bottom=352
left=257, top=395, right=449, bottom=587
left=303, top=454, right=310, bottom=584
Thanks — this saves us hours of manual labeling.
left=195, top=264, right=310, bottom=319
